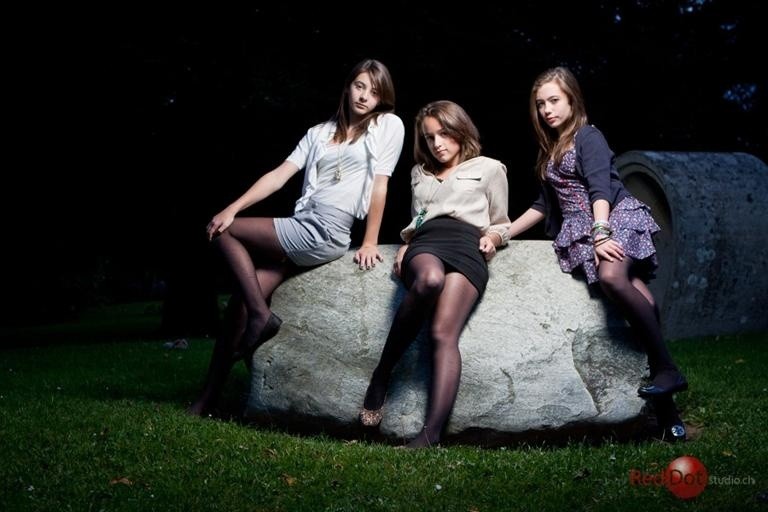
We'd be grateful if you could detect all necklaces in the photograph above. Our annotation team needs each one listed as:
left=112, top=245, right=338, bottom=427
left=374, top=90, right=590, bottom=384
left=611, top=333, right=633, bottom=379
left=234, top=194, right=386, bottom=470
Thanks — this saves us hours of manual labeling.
left=334, top=120, right=354, bottom=186
left=414, top=165, right=453, bottom=228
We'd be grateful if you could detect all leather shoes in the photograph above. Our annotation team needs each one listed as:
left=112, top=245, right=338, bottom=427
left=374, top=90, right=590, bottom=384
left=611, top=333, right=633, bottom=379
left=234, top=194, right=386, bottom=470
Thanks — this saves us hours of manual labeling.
left=227, top=314, right=282, bottom=363
left=637, top=374, right=690, bottom=397
left=360, top=388, right=388, bottom=427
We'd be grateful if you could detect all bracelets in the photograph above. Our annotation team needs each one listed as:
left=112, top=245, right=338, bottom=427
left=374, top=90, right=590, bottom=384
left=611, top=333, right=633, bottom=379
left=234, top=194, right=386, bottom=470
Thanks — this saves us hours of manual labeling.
left=587, top=218, right=613, bottom=249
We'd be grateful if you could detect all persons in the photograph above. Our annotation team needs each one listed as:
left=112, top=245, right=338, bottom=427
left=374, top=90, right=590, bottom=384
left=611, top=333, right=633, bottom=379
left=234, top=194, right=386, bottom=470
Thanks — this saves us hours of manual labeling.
left=355, top=97, right=514, bottom=452
left=506, top=66, right=692, bottom=444
left=183, top=59, right=408, bottom=417
